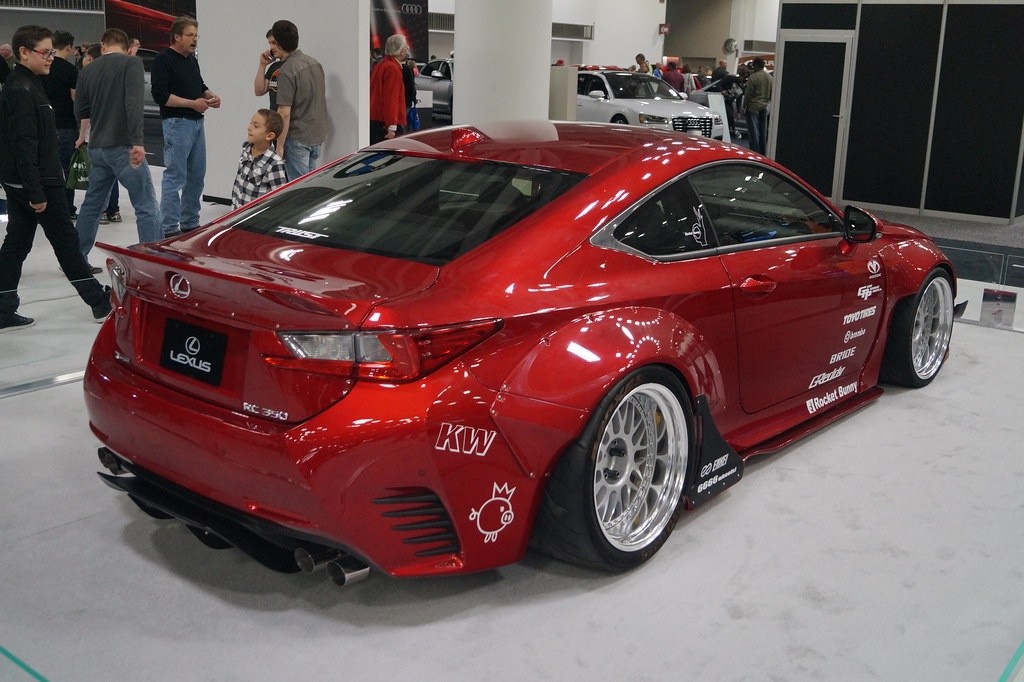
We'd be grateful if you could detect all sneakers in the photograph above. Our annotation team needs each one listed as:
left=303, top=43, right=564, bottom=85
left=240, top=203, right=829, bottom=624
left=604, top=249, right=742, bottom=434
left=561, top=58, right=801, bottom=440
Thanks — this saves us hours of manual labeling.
left=92, top=285, right=114, bottom=322
left=0, top=311, right=35, bottom=332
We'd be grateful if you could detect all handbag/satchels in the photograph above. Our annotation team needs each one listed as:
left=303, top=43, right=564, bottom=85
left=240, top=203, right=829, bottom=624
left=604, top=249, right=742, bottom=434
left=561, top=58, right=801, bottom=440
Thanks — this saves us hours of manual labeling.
left=408, top=102, right=421, bottom=131
left=686, top=88, right=695, bottom=99
left=65, top=143, right=91, bottom=190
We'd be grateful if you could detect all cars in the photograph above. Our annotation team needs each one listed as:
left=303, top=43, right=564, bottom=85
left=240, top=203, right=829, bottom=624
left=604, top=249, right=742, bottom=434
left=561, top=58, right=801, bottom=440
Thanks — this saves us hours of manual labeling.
left=575, top=71, right=724, bottom=141
left=690, top=74, right=748, bottom=127
left=417, top=58, right=453, bottom=115
left=136, top=48, right=160, bottom=116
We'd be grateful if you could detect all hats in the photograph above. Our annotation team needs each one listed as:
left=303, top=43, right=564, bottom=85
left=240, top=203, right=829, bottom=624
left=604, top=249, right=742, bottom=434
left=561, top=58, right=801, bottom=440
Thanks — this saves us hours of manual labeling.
left=75, top=46, right=84, bottom=57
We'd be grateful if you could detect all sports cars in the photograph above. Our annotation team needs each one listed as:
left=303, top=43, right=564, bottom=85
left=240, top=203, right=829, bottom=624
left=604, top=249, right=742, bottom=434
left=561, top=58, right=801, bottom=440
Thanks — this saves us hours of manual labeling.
left=80, top=120, right=957, bottom=590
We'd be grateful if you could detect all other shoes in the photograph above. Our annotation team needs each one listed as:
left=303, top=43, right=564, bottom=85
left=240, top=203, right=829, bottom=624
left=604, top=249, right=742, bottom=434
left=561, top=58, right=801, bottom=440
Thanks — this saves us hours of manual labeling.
left=59, top=257, right=103, bottom=274
left=108, top=211, right=122, bottom=222
left=70, top=212, right=79, bottom=219
left=99, top=212, right=109, bottom=224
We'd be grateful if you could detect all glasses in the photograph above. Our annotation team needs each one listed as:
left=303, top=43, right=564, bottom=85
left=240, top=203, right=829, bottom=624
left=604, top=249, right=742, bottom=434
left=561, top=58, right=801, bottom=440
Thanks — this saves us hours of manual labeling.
left=402, top=45, right=409, bottom=50
left=24, top=46, right=57, bottom=59
left=181, top=33, right=200, bottom=40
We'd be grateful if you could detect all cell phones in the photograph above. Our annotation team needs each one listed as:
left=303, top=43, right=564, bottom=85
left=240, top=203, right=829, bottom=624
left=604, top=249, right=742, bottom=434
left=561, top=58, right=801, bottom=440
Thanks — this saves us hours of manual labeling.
left=270, top=51, right=275, bottom=60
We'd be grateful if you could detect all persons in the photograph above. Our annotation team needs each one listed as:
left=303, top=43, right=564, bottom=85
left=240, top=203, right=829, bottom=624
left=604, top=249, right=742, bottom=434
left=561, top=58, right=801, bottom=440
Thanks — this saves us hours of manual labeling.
left=742, top=56, right=773, bottom=156
left=0, top=25, right=113, bottom=333
left=151, top=17, right=222, bottom=239
left=254, top=20, right=333, bottom=182
left=0, top=29, right=123, bottom=225
left=59, top=28, right=165, bottom=274
left=370, top=34, right=419, bottom=148
left=230, top=109, right=289, bottom=210
left=627, top=54, right=748, bottom=130
